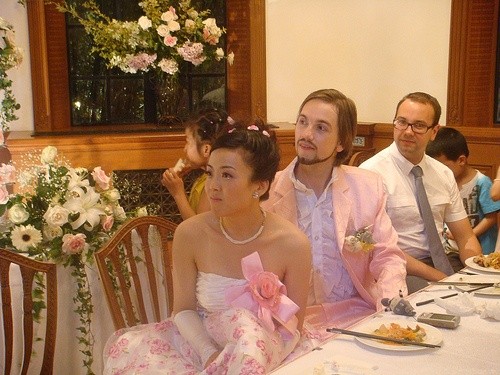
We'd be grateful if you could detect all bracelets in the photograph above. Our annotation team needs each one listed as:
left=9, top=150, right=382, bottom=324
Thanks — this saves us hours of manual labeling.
left=492, top=178, right=500, bottom=183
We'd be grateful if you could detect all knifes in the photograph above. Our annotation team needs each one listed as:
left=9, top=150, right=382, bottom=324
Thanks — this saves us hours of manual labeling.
left=427, top=282, right=494, bottom=286
left=457, top=271, right=478, bottom=275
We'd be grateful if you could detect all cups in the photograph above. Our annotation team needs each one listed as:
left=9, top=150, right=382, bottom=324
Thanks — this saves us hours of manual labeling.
left=162, top=157, right=195, bottom=187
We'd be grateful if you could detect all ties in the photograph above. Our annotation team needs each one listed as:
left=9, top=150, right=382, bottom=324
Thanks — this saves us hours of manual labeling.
left=411, top=166, right=454, bottom=277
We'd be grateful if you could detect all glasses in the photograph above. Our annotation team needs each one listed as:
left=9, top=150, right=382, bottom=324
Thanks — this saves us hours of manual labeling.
left=393, top=116, right=435, bottom=135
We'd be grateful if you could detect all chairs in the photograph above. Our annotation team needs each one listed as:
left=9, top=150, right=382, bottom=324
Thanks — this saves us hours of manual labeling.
left=93, top=217, right=178, bottom=329
left=0, top=249, right=57, bottom=375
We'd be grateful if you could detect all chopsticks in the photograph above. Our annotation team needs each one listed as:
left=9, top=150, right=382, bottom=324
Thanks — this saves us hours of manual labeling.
left=326, top=328, right=442, bottom=349
left=416, top=286, right=492, bottom=306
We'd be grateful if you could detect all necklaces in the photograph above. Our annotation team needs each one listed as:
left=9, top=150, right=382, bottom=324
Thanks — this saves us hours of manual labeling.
left=219, top=205, right=267, bottom=244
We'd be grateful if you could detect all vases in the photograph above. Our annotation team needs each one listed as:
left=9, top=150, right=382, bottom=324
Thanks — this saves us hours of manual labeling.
left=152, top=69, right=180, bottom=124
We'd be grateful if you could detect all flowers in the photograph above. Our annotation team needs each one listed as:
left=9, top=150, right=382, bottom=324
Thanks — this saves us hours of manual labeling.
left=340, top=225, right=377, bottom=254
left=0, top=145, right=160, bottom=375
left=46, top=0, right=236, bottom=78
left=0, top=18, right=25, bottom=132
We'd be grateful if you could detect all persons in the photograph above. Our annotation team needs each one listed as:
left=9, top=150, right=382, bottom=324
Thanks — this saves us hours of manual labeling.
left=259, top=88, right=408, bottom=349
left=161, top=109, right=236, bottom=221
left=358, top=91, right=482, bottom=294
left=426, top=126, right=500, bottom=273
left=102, top=118, right=312, bottom=375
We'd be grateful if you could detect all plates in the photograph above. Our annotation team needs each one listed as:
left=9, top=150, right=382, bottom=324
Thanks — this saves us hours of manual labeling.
left=454, top=274, right=500, bottom=295
left=464, top=255, right=500, bottom=273
left=353, top=320, right=443, bottom=351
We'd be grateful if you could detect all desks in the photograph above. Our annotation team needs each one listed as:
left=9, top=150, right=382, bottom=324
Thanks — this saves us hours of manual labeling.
left=265, top=265, right=500, bottom=375
left=0, top=224, right=168, bottom=375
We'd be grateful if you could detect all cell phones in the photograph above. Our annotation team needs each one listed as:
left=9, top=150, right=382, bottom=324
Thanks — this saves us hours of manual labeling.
left=417, top=312, right=460, bottom=327
